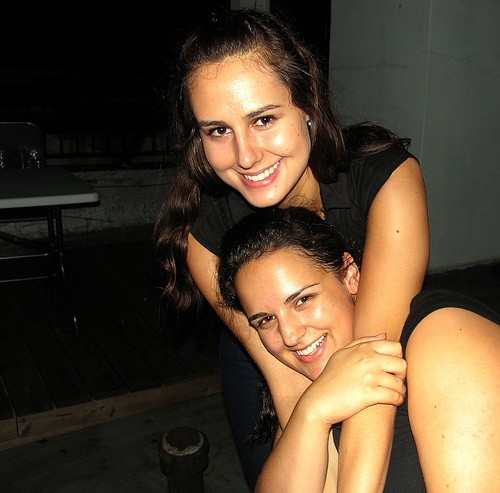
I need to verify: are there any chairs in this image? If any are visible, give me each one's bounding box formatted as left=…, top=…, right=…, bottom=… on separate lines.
left=0, top=122, right=80, bottom=308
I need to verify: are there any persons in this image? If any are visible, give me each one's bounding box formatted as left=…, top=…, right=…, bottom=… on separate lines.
left=214, top=206, right=500, bottom=492
left=152, top=7, right=429, bottom=493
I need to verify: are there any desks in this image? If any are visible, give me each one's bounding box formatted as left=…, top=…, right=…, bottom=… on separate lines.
left=0, top=166, right=99, bottom=342
